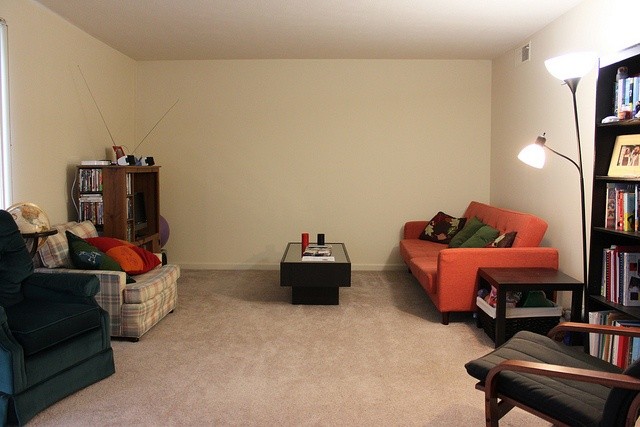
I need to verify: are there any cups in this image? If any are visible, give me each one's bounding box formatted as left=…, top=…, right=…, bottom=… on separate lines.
left=317, top=233, right=325, bottom=244
left=301, top=233, right=310, bottom=253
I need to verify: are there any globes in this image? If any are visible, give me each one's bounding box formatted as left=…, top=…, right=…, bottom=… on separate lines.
left=6, top=202, right=58, bottom=260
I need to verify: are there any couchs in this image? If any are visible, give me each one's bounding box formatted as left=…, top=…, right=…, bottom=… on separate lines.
left=399, top=199, right=560, bottom=324
left=26, top=220, right=181, bottom=341
left=0, top=210, right=116, bottom=427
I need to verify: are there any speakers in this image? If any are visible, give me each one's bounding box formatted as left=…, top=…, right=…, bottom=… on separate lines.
left=117, top=155, right=136, bottom=166
left=141, top=156, right=155, bottom=166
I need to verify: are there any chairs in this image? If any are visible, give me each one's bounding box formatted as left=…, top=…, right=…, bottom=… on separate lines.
left=465, top=321, right=640, bottom=427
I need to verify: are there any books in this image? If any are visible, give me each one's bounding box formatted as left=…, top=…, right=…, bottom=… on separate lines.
left=125, top=173, right=132, bottom=195
left=605, top=181, right=639, bottom=234
left=79, top=169, right=102, bottom=192
left=587, top=311, right=640, bottom=369
left=126, top=197, right=132, bottom=221
left=613, top=76, right=640, bottom=118
left=601, top=245, right=640, bottom=306
left=78, top=194, right=104, bottom=226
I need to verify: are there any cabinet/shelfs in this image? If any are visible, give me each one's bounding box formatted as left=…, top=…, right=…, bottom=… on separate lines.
left=75, top=163, right=161, bottom=256
left=583, top=41, right=638, bottom=362
left=476, top=267, right=584, bottom=349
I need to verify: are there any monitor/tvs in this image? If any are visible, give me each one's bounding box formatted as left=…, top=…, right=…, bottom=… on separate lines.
left=133, top=191, right=148, bottom=240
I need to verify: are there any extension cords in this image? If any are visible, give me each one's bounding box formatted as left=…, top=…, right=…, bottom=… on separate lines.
left=80, top=160, right=112, bottom=166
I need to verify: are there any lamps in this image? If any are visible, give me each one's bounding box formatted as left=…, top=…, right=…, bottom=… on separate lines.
left=514, top=49, right=599, bottom=318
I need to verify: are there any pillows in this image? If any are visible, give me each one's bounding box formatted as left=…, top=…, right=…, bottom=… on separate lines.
left=460, top=226, right=500, bottom=248
left=35, top=219, right=99, bottom=268
left=418, top=210, right=467, bottom=244
left=447, top=216, right=485, bottom=247
left=485, top=231, right=517, bottom=247
left=66, top=229, right=135, bottom=283
left=82, top=234, right=163, bottom=272
